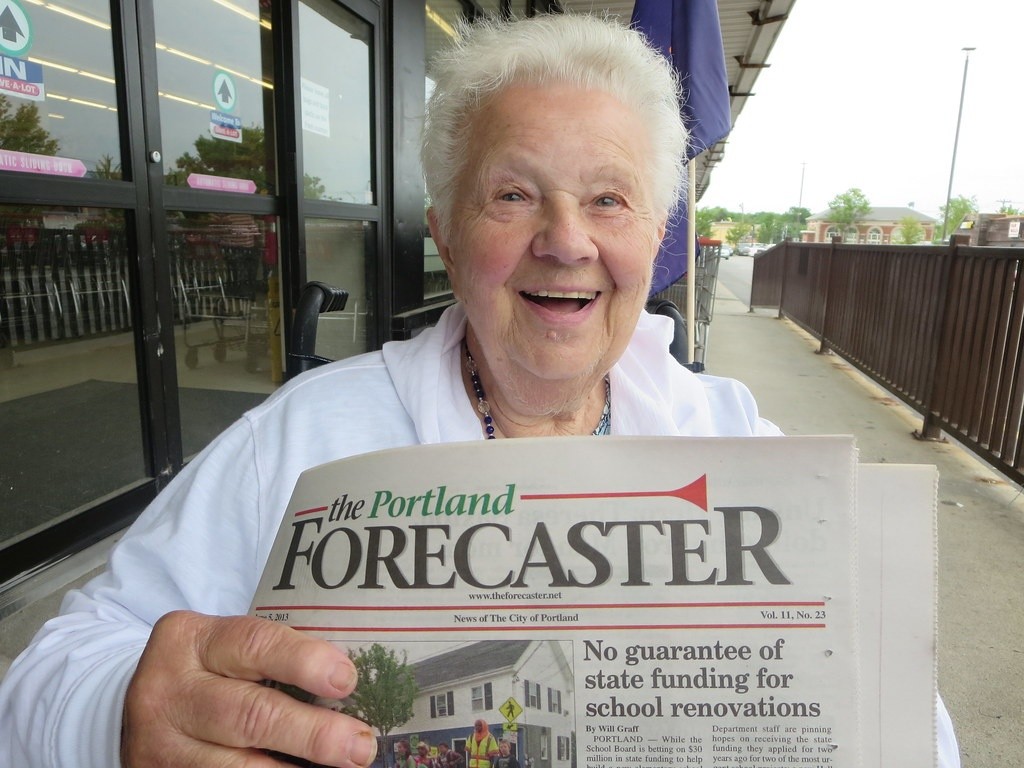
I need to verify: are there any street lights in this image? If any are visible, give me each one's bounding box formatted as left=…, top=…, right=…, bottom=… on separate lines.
left=795, top=162, right=811, bottom=236
left=940, top=46, right=977, bottom=241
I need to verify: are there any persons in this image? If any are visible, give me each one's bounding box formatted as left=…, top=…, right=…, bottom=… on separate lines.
left=464, top=720, right=500, bottom=768
left=395, top=740, right=428, bottom=768
left=434, top=743, right=464, bottom=768
left=493, top=739, right=521, bottom=768
left=208, top=213, right=259, bottom=302
left=0, top=13, right=958, bottom=768
left=416, top=742, right=436, bottom=768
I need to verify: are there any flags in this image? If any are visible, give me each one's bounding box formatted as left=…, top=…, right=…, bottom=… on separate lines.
left=628, top=0, right=731, bottom=301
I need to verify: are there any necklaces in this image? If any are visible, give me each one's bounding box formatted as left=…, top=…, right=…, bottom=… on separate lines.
left=466, top=344, right=495, bottom=440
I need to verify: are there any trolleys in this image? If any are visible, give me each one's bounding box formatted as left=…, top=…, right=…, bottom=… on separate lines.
left=648, top=237, right=722, bottom=373
left=289, top=278, right=697, bottom=377
left=169, top=255, right=271, bottom=374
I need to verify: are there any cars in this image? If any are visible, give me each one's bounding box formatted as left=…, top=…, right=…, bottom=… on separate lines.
left=714, top=241, right=777, bottom=260
